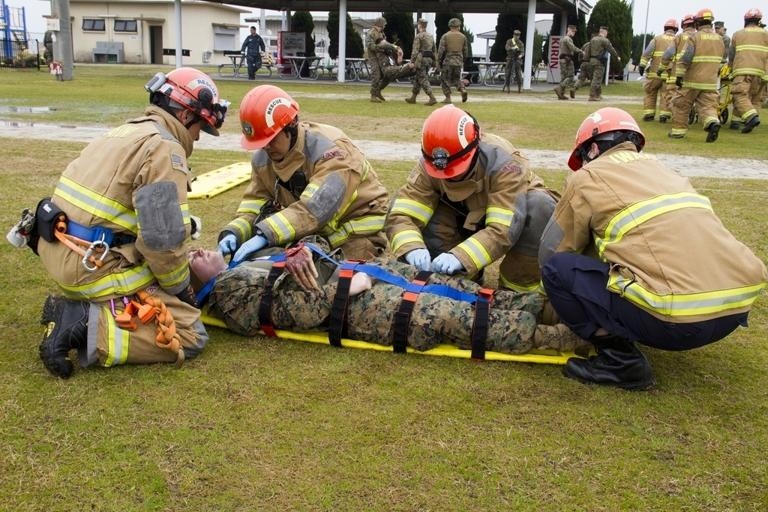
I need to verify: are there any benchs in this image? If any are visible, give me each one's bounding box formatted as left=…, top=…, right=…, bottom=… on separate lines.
left=216, top=52, right=508, bottom=88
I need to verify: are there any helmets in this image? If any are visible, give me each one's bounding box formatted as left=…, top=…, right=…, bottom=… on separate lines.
left=448, top=18, right=461, bottom=27
left=375, top=17, right=386, bottom=27
left=421, top=104, right=481, bottom=180
left=238, top=85, right=299, bottom=150
left=664, top=8, right=714, bottom=32
left=744, top=8, right=762, bottom=19
left=567, top=107, right=645, bottom=171
left=150, top=67, right=221, bottom=137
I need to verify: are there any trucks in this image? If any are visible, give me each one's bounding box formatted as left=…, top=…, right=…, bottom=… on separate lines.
left=259, top=37, right=279, bottom=64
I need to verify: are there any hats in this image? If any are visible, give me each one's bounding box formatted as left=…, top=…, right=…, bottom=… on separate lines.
left=715, top=22, right=724, bottom=27
left=417, top=18, right=428, bottom=27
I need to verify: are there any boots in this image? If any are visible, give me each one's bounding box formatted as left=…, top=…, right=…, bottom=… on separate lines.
left=38, top=294, right=90, bottom=380
left=405, top=93, right=417, bottom=104
left=570, top=87, right=577, bottom=98
left=706, top=123, right=720, bottom=142
left=460, top=88, right=467, bottom=102
left=563, top=332, right=657, bottom=392
left=425, top=95, right=437, bottom=106
left=441, top=94, right=451, bottom=103
left=554, top=86, right=567, bottom=99
left=741, top=116, right=760, bottom=134
left=371, top=94, right=381, bottom=102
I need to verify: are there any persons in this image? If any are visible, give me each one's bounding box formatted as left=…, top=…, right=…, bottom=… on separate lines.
left=241, top=26, right=265, bottom=80
left=554, top=25, right=584, bottom=100
left=187, top=239, right=594, bottom=354
left=365, top=18, right=391, bottom=103
left=585, top=26, right=621, bottom=101
left=375, top=38, right=417, bottom=81
left=639, top=8, right=768, bottom=143
left=438, top=18, right=468, bottom=104
left=405, top=19, right=437, bottom=106
left=217, top=86, right=390, bottom=267
left=570, top=34, right=597, bottom=98
left=15, top=66, right=230, bottom=380
left=505, top=30, right=525, bottom=93
left=539, top=108, right=766, bottom=392
left=385, top=103, right=558, bottom=293
left=428, top=76, right=470, bottom=87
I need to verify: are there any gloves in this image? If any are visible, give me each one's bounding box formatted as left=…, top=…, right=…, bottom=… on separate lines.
left=639, top=66, right=644, bottom=77
left=233, top=234, right=268, bottom=263
left=431, top=252, right=463, bottom=275
left=218, top=234, right=237, bottom=255
left=405, top=248, right=431, bottom=272
left=657, top=70, right=663, bottom=77
left=676, top=76, right=684, bottom=87
